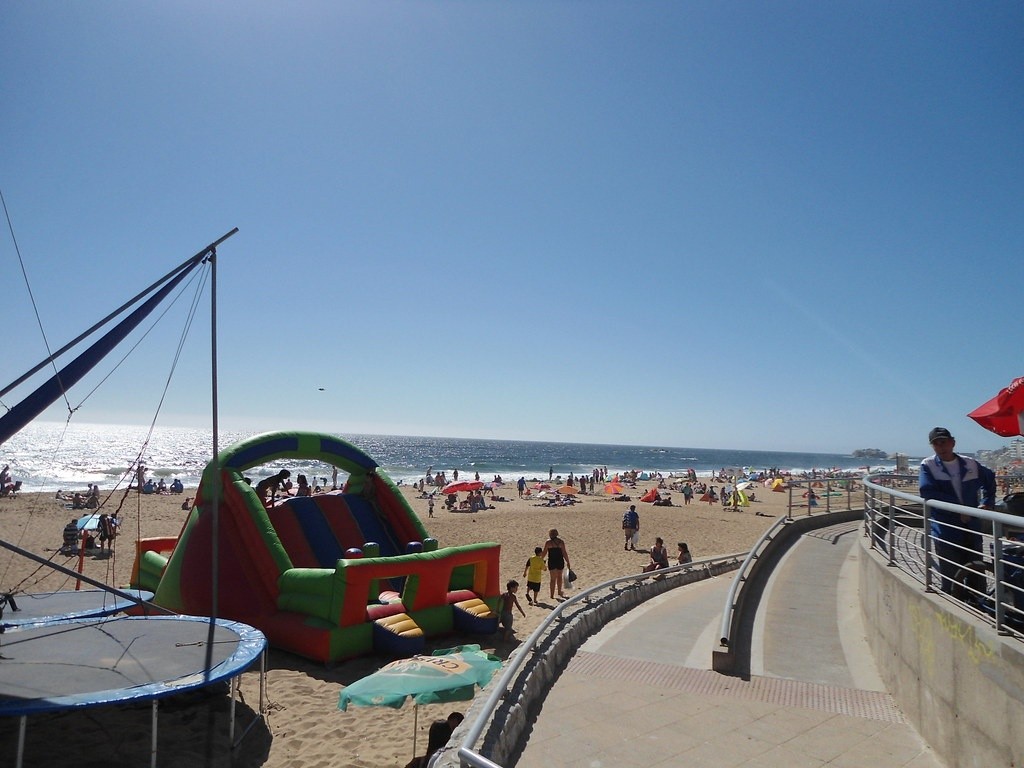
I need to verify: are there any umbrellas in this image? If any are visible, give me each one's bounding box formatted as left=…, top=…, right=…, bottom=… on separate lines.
left=611, top=476, right=619, bottom=483
left=76, top=514, right=118, bottom=532
left=559, top=486, right=578, bottom=497
left=966, top=376, right=1024, bottom=437
left=765, top=478, right=773, bottom=487
left=535, top=484, right=551, bottom=489
left=442, top=480, right=469, bottom=502
left=641, top=487, right=658, bottom=502
left=604, top=483, right=624, bottom=493
left=459, top=481, right=484, bottom=492
left=858, top=466, right=866, bottom=469
left=732, top=482, right=751, bottom=491
left=338, top=644, right=503, bottom=760
left=771, top=479, right=782, bottom=488
left=674, top=478, right=689, bottom=483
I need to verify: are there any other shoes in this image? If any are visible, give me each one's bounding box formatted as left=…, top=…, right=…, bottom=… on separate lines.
left=625, top=543, right=634, bottom=551
left=526, top=594, right=532, bottom=602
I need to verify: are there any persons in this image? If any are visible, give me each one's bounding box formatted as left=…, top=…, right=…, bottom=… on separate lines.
left=63, top=513, right=120, bottom=555
left=497, top=580, right=527, bottom=642
left=427, top=712, right=465, bottom=761
left=650, top=537, right=670, bottom=575
left=428, top=494, right=435, bottom=518
left=676, top=542, right=693, bottom=572
left=540, top=528, right=570, bottom=599
left=395, top=463, right=1024, bottom=512
left=136, top=466, right=183, bottom=494
left=182, top=498, right=191, bottom=510
left=622, top=505, right=639, bottom=550
left=55, top=483, right=100, bottom=509
left=245, top=465, right=345, bottom=510
left=919, top=427, right=997, bottom=612
left=0, top=466, right=10, bottom=492
left=523, top=547, right=547, bottom=602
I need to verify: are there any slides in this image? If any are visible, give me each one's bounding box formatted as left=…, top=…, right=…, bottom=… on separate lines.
left=264, top=491, right=408, bottom=593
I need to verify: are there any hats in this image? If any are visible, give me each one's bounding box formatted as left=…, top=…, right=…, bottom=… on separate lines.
left=928, top=426, right=953, bottom=443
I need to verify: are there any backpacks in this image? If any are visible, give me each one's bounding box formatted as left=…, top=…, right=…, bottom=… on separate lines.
left=622, top=511, right=637, bottom=528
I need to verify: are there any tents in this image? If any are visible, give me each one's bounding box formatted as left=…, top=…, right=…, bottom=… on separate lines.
left=637, top=472, right=648, bottom=480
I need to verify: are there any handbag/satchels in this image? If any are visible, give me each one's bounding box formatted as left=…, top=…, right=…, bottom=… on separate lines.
left=563, top=566, right=577, bottom=589
left=632, top=530, right=639, bottom=544
